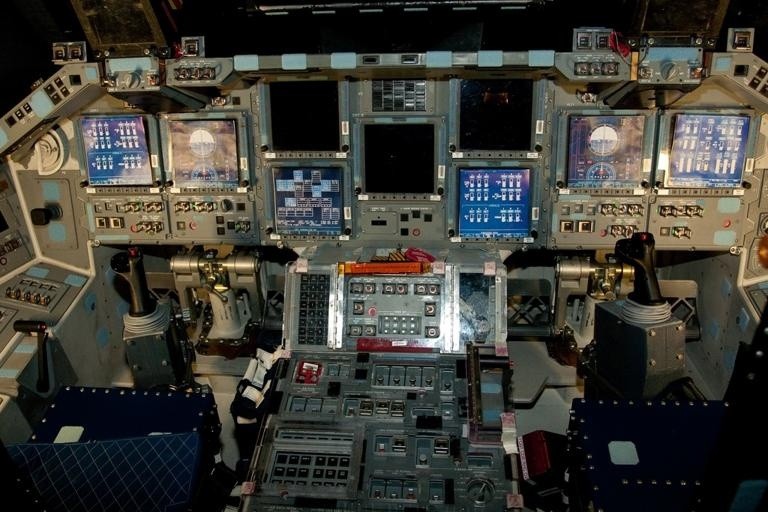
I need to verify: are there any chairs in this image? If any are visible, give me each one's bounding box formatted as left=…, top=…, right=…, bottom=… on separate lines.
left=562, top=302, right=768, bottom=511
left=0, top=382, right=235, bottom=511
